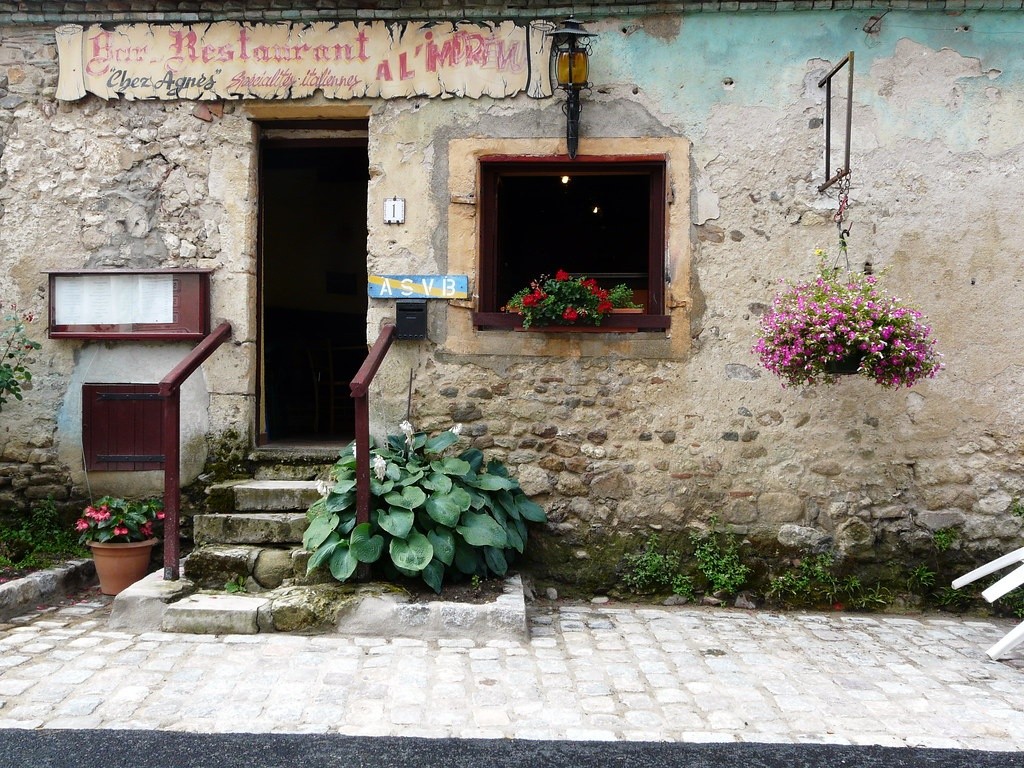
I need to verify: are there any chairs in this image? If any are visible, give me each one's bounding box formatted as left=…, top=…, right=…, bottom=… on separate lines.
left=304, top=336, right=352, bottom=433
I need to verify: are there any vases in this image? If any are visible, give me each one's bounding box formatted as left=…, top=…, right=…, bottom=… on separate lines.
left=82, top=538, right=159, bottom=596
left=821, top=337, right=865, bottom=376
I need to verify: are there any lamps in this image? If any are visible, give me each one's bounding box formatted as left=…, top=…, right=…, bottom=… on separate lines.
left=544, top=14, right=598, bottom=158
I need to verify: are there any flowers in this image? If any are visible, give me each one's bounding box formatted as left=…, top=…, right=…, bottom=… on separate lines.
left=499, top=268, right=636, bottom=329
left=75, top=495, right=168, bottom=544
left=749, top=250, right=945, bottom=393
